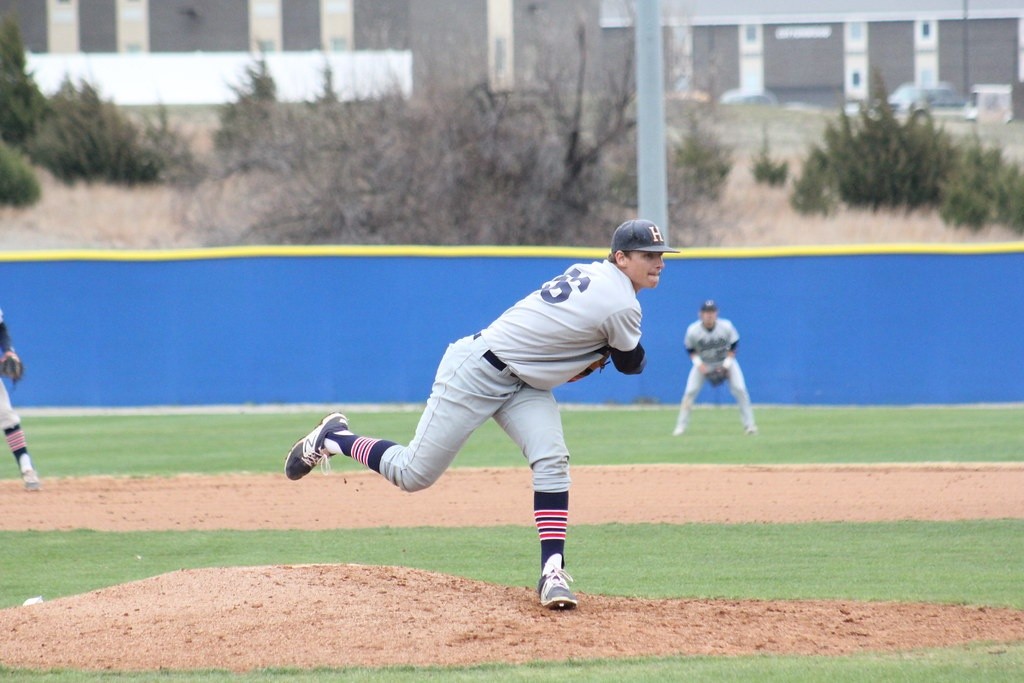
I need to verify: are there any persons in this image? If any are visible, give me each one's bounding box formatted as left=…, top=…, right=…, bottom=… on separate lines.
left=0, top=309, right=40, bottom=491
left=284, top=220, right=680, bottom=609
left=672, top=300, right=759, bottom=436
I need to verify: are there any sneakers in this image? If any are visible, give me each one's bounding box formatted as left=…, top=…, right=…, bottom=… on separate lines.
left=284, top=412, right=348, bottom=481
left=538, top=560, right=577, bottom=609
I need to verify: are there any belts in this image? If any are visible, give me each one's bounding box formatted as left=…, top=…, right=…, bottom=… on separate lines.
left=473, top=332, right=518, bottom=378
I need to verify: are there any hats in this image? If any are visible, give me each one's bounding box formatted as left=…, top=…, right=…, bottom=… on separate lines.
left=610, top=219, right=681, bottom=253
left=702, top=301, right=716, bottom=312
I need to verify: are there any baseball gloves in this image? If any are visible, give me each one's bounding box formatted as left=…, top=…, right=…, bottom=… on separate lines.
left=704, top=368, right=730, bottom=386
left=567, top=349, right=610, bottom=382
left=0, top=351, right=24, bottom=381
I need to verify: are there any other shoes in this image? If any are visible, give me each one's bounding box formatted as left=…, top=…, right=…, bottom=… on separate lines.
left=21, top=469, right=41, bottom=491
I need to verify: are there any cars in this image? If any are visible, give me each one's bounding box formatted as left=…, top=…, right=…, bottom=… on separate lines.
left=887, top=82, right=966, bottom=115
left=720, top=89, right=778, bottom=108
